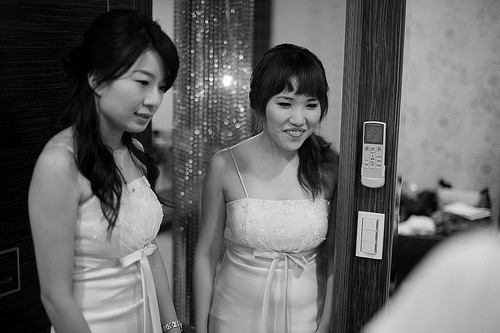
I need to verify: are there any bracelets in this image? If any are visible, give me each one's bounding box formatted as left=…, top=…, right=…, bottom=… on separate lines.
left=163, top=321, right=183, bottom=332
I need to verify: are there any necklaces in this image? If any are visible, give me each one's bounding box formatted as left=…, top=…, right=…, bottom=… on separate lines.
left=110, top=143, right=135, bottom=192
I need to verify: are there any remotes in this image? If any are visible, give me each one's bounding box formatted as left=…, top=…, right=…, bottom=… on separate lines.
left=361, top=121, right=387, bottom=188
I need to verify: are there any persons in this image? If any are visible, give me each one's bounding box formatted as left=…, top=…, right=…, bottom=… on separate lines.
left=28, top=7, right=182, bottom=333
left=192, top=43, right=339, bottom=333
